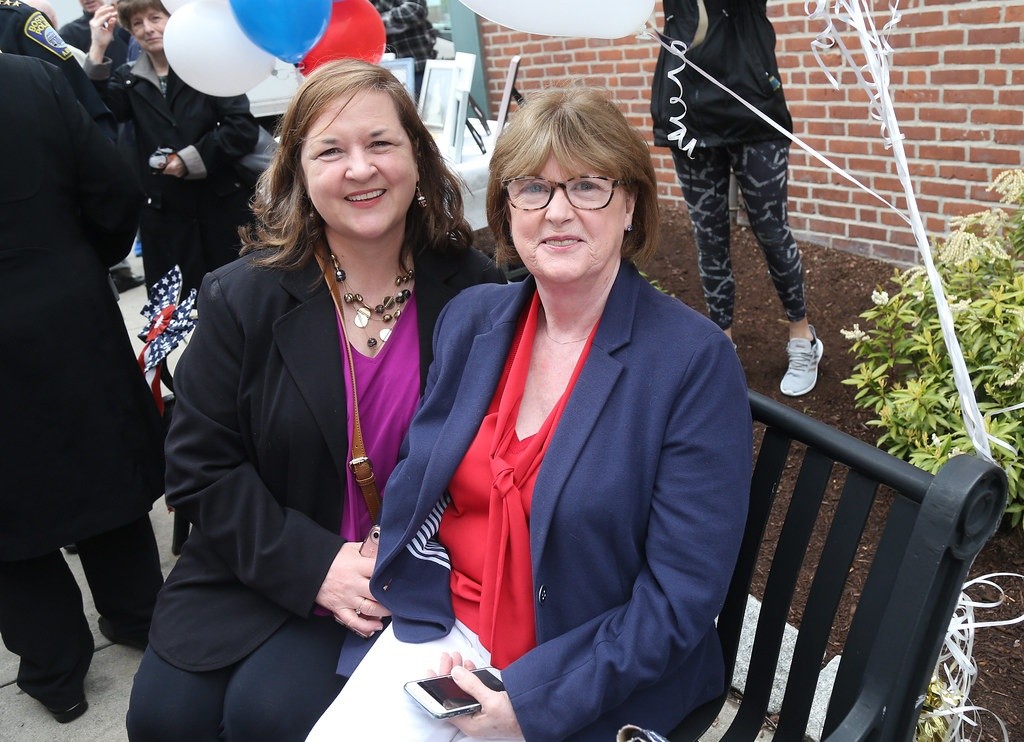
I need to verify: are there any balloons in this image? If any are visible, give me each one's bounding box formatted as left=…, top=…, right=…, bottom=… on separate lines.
left=459, top=1, right=658, bottom=39
left=163, top=0, right=386, bottom=98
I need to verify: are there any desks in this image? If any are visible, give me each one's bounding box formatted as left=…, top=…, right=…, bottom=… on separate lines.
left=439, top=119, right=511, bottom=228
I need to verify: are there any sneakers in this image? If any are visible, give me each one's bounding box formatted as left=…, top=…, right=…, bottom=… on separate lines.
left=780, top=325, right=823, bottom=396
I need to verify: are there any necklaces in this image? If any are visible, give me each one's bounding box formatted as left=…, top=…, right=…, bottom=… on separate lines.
left=331, top=252, right=414, bottom=350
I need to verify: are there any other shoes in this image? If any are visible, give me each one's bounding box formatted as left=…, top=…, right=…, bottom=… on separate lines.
left=48, top=692, right=88, bottom=724
left=98, top=614, right=148, bottom=651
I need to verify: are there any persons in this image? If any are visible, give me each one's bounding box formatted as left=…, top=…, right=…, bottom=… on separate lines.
left=0, top=0, right=119, bottom=147
left=84, top=0, right=260, bottom=308
left=58, top=0, right=131, bottom=71
left=0, top=53, right=167, bottom=723
left=305, top=90, right=751, bottom=742
left=127, top=61, right=506, bottom=742
left=649, top=0, right=823, bottom=397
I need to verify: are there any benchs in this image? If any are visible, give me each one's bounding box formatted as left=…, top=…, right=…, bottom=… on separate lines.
left=160, top=359, right=1009, bottom=742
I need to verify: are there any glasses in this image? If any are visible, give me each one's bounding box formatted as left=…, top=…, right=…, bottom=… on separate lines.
left=501, top=173, right=634, bottom=211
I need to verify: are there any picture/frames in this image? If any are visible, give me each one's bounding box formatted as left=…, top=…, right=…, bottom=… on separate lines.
left=381, top=50, right=476, bottom=163
left=496, top=55, right=523, bottom=137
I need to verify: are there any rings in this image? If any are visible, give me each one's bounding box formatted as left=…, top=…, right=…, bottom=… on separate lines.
left=356, top=599, right=365, bottom=614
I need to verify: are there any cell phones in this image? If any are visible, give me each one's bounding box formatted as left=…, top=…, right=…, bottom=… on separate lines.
left=405, top=667, right=507, bottom=718
left=335, top=526, right=383, bottom=638
